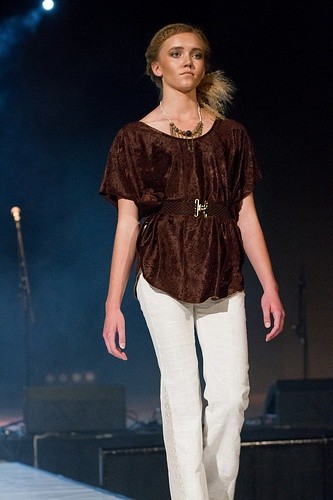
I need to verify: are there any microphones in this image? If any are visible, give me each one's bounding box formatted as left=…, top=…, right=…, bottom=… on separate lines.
left=11, top=206, right=20, bottom=222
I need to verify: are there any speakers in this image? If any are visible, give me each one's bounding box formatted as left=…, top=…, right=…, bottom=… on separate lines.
left=23, top=383, right=126, bottom=435
left=262, top=378, right=333, bottom=428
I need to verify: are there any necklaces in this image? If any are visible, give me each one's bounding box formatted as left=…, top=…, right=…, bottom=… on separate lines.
left=159, top=98, right=206, bottom=138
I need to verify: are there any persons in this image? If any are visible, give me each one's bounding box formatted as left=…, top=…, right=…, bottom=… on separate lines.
left=99, top=21, right=287, bottom=499
